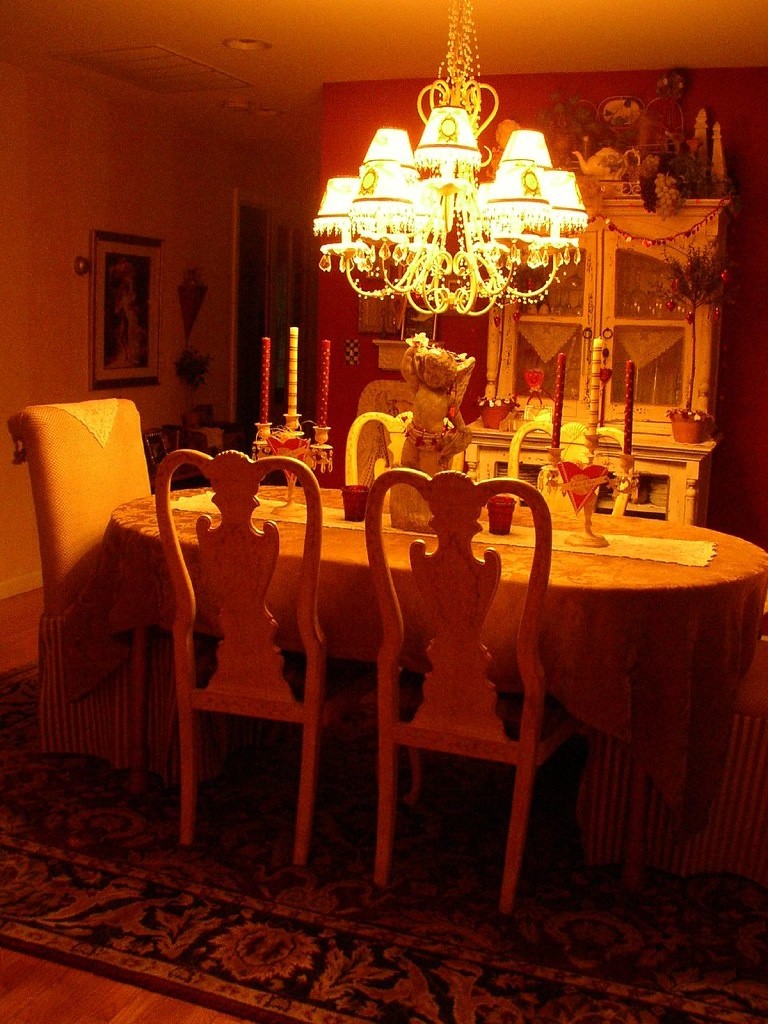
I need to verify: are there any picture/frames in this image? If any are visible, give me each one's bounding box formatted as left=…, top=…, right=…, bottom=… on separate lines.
left=146, top=427, right=166, bottom=468
left=89, top=229, right=163, bottom=389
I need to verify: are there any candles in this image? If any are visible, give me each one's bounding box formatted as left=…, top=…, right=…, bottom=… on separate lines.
left=252, top=321, right=333, bottom=428
left=591, top=332, right=603, bottom=435
left=623, top=360, right=637, bottom=455
left=553, top=350, right=567, bottom=448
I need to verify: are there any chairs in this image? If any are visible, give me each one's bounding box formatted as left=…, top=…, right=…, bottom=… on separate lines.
left=507, top=419, right=636, bottom=515
left=6, top=399, right=152, bottom=793
left=345, top=404, right=428, bottom=515
left=580, top=609, right=766, bottom=888
left=366, top=472, right=563, bottom=912
left=132, top=449, right=318, bottom=865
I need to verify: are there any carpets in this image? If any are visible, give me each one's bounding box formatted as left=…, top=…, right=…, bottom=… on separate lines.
left=0, top=627, right=767, bottom=1023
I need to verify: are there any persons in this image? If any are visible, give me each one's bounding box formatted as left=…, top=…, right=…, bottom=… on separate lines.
left=400, top=345, right=472, bottom=477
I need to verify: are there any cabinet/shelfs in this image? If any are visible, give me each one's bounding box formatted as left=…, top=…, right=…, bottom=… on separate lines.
left=474, top=435, right=720, bottom=519
left=487, top=197, right=730, bottom=436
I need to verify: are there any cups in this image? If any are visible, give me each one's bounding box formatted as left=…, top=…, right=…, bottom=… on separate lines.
left=340, top=485, right=369, bottom=522
left=487, top=495, right=516, bottom=535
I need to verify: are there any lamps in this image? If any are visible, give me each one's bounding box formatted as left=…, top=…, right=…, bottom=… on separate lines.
left=311, top=0, right=589, bottom=317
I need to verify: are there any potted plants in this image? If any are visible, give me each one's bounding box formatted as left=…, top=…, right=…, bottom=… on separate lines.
left=176, top=348, right=214, bottom=427
left=667, top=226, right=728, bottom=442
left=483, top=229, right=520, bottom=431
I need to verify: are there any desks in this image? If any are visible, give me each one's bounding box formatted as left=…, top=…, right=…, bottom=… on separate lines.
left=101, top=483, right=766, bottom=882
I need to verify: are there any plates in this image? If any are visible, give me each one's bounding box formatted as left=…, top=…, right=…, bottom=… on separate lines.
left=496, top=463, right=540, bottom=489
left=599, top=474, right=667, bottom=509
